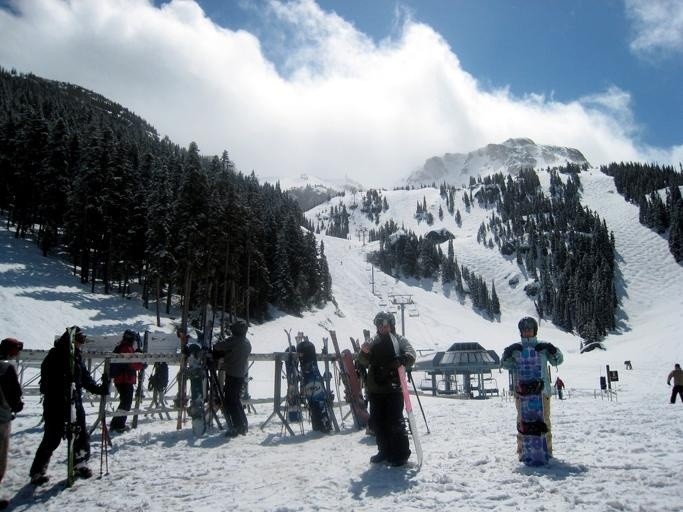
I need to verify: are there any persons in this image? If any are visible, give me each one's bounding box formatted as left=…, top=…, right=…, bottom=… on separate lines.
left=357, top=312, right=416, bottom=468
left=0, top=338, right=25, bottom=510
left=667, top=363, right=683, bottom=404
left=29, top=325, right=109, bottom=485
left=500, top=317, right=564, bottom=462
left=102, top=328, right=148, bottom=433
left=553, top=376, right=566, bottom=400
left=213, top=321, right=251, bottom=437
left=152, top=362, right=169, bottom=409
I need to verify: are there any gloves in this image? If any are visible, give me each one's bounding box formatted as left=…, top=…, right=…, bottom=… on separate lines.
left=393, top=354, right=410, bottom=367
left=98, top=383, right=108, bottom=394
left=64, top=390, right=77, bottom=403
left=535, top=342, right=555, bottom=354
left=504, top=343, right=521, bottom=357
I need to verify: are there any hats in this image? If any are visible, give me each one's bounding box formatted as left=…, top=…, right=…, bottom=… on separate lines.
left=123, top=329, right=138, bottom=345
left=230, top=320, right=247, bottom=335
left=63, top=326, right=83, bottom=346
left=0, top=339, right=18, bottom=357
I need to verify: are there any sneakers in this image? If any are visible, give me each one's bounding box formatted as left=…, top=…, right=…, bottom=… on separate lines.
left=226, top=424, right=248, bottom=436
left=110, top=425, right=129, bottom=433
left=32, top=473, right=48, bottom=485
left=370, top=449, right=407, bottom=465
left=73, top=466, right=91, bottom=478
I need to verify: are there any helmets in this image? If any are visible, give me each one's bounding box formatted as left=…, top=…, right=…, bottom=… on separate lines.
left=518, top=317, right=537, bottom=335
left=375, top=311, right=394, bottom=327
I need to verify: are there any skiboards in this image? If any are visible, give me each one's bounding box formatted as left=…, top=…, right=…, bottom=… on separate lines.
left=131, top=331, right=148, bottom=428
left=280, top=329, right=370, bottom=436
left=67, top=326, right=78, bottom=488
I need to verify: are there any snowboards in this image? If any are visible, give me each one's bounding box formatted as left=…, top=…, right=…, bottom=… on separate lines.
left=515, top=343, right=549, bottom=467
left=389, top=332, right=423, bottom=470
left=187, top=303, right=214, bottom=436
